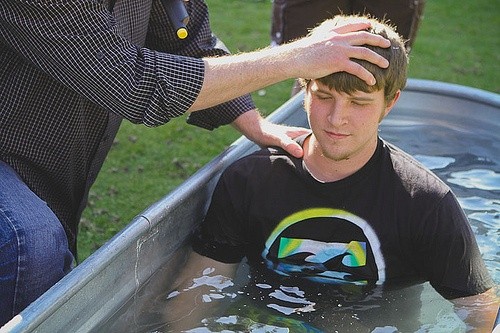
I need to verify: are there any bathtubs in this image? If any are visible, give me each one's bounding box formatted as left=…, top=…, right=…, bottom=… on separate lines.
left=0, top=75, right=499, bottom=333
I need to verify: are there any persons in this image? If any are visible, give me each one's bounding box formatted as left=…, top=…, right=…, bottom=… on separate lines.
left=0, top=0, right=393, bottom=328
left=164, top=13, right=500, bottom=333
